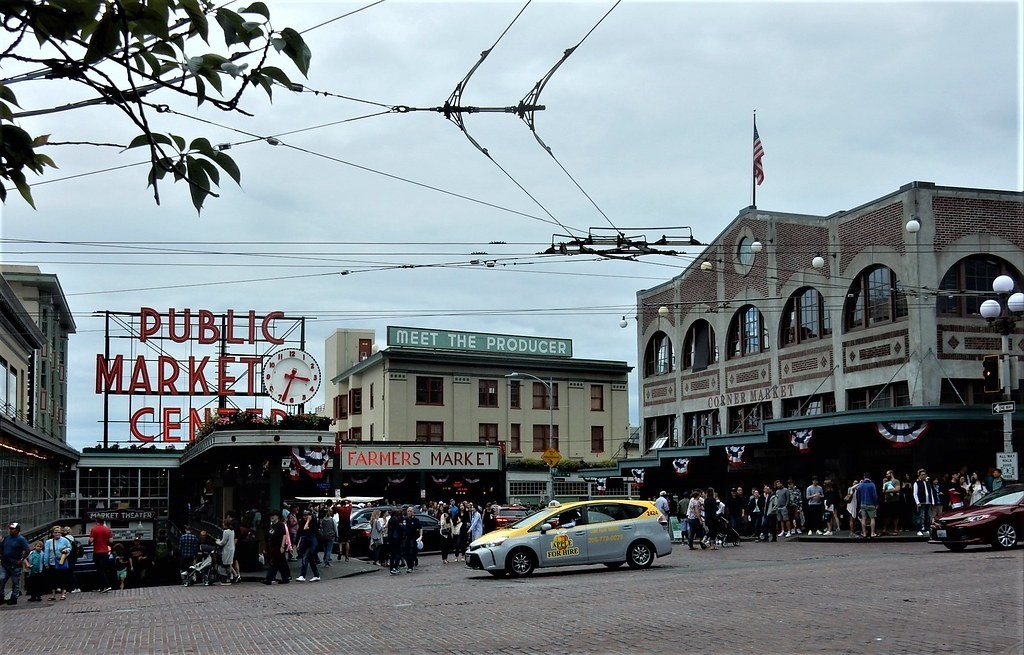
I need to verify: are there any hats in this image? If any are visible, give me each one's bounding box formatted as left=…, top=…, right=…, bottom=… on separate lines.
left=8, top=523, right=20, bottom=532
left=302, top=509, right=313, bottom=515
left=660, top=491, right=668, bottom=495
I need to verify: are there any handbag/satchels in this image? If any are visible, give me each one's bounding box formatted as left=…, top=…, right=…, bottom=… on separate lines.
left=452, top=522, right=463, bottom=535
left=844, top=490, right=856, bottom=503
left=55, top=557, right=68, bottom=569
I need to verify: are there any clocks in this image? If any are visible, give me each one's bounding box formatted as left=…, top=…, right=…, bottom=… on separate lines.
left=262, top=348, right=321, bottom=405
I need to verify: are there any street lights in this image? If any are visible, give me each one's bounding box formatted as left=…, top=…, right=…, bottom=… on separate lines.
left=504, top=371, right=553, bottom=502
left=980, top=275, right=1024, bottom=452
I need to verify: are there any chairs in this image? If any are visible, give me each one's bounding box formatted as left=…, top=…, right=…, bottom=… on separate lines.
left=569, top=509, right=579, bottom=520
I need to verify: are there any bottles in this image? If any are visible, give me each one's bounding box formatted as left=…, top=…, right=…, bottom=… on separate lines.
left=59, top=552, right=66, bottom=565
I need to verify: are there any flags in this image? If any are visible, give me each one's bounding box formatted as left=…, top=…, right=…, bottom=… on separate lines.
left=753, top=115, right=765, bottom=186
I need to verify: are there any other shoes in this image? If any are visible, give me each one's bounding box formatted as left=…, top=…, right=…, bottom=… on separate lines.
left=682, top=527, right=931, bottom=551
left=28, top=598, right=36, bottom=602
left=60, top=597, right=66, bottom=600
left=48, top=598, right=56, bottom=601
left=36, top=598, right=41, bottom=601
left=92, top=588, right=101, bottom=592
left=7, top=598, right=17, bottom=605
left=220, top=556, right=466, bottom=586
left=0, top=596, right=4, bottom=605
left=102, top=586, right=111, bottom=592
left=71, top=588, right=81, bottom=594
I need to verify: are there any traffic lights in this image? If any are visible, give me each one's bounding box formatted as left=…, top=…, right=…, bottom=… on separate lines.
left=982, top=355, right=1000, bottom=392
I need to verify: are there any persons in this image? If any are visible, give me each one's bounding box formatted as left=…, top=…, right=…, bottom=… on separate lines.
left=1, top=494, right=544, bottom=607
left=657, top=469, right=1004, bottom=551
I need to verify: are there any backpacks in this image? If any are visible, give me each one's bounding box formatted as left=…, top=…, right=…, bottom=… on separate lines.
left=65, top=536, right=84, bottom=558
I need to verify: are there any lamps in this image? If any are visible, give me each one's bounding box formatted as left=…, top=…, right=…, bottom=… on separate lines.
left=906, top=215, right=922, bottom=234
left=619, top=315, right=639, bottom=328
left=812, top=251, right=837, bottom=267
left=701, top=257, right=722, bottom=272
left=751, top=238, right=774, bottom=253
left=658, top=303, right=679, bottom=317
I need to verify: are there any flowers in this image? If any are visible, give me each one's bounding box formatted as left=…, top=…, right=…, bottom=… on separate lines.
left=215, top=411, right=330, bottom=425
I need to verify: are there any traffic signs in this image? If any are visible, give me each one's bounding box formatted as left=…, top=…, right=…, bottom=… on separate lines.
left=992, top=401, right=1016, bottom=415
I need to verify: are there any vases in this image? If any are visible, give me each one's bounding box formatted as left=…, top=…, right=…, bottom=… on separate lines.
left=216, top=424, right=329, bottom=431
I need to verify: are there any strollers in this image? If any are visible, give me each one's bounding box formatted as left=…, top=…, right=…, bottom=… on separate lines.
left=179, top=544, right=223, bottom=586
left=716, top=512, right=744, bottom=548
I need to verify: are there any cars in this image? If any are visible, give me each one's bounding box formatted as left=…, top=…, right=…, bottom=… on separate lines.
left=929, top=483, right=1024, bottom=551
left=491, top=504, right=530, bottom=529
left=72, top=545, right=115, bottom=589
left=342, top=506, right=441, bottom=552
left=465, top=500, right=673, bottom=579
